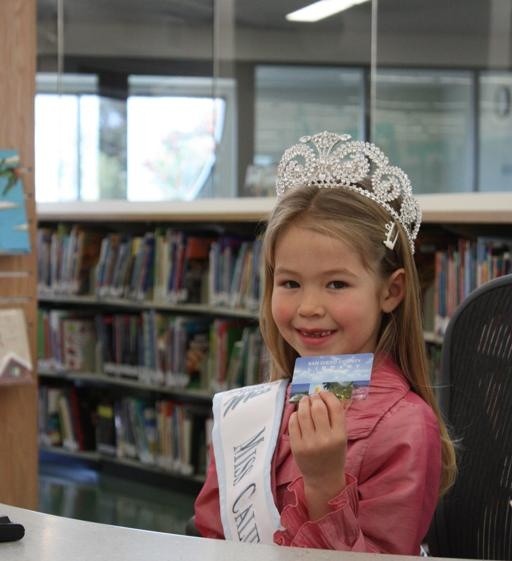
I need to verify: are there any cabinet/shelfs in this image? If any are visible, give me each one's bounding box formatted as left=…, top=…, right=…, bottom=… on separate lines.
left=38, top=193, right=512, bottom=498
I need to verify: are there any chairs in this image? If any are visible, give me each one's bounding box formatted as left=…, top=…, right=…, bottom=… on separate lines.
left=430, top=272, right=510, bottom=558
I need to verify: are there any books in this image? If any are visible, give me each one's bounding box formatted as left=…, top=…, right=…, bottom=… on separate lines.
left=424, top=235, right=512, bottom=395
left=36, top=225, right=281, bottom=484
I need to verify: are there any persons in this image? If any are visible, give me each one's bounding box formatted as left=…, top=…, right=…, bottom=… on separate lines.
left=193, top=179, right=454, bottom=558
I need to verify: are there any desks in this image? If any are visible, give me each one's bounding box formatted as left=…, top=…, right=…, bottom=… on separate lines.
left=0, top=497, right=418, bottom=559
